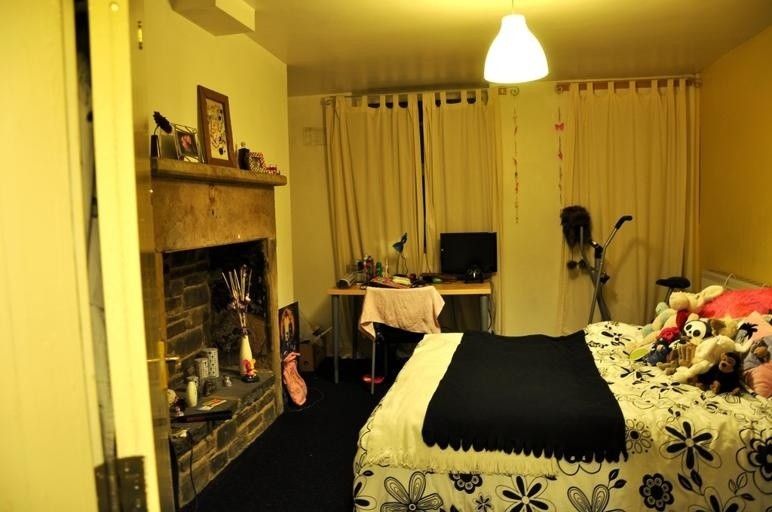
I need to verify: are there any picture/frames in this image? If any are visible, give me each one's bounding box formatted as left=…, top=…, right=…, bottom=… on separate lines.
left=197, top=85, right=237, bottom=167
left=176, top=131, right=200, bottom=161
left=249, top=152, right=265, bottom=172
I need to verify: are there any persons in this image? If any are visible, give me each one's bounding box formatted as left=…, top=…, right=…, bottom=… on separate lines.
left=181, top=132, right=196, bottom=153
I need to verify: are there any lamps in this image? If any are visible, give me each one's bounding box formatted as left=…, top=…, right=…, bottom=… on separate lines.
left=482, top=1, right=549, bottom=84
left=394, top=234, right=409, bottom=279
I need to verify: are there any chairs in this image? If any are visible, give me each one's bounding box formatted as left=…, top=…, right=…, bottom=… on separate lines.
left=365, top=285, right=436, bottom=395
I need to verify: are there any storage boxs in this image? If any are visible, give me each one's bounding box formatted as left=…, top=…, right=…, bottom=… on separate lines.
left=299, top=340, right=324, bottom=372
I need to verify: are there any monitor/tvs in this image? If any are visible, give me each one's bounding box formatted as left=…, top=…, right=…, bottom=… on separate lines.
left=440, top=232, right=498, bottom=284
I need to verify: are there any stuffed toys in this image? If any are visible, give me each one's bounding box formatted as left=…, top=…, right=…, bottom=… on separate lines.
left=642, top=337, right=672, bottom=366
left=656, top=313, right=738, bottom=375
left=693, top=352, right=742, bottom=395
left=626, top=285, right=723, bottom=361
left=672, top=316, right=753, bottom=382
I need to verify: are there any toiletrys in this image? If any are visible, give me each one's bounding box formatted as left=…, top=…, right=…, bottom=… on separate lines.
left=376, top=262, right=381, bottom=275
left=385, top=258, right=389, bottom=277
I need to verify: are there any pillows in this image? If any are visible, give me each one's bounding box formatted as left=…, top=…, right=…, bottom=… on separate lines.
left=732, top=311, right=772, bottom=398
left=350, top=322, right=772, bottom=512
left=700, top=288, right=772, bottom=319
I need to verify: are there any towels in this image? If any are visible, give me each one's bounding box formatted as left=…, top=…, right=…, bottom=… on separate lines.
left=357, top=286, right=445, bottom=342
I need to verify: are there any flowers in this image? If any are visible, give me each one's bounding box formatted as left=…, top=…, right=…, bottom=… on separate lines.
left=151, top=111, right=172, bottom=135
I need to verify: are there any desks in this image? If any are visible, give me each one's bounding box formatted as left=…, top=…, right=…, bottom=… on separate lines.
left=328, top=277, right=490, bottom=385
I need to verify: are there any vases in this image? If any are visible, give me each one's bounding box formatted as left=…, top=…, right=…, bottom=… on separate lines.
left=239, top=143, right=249, bottom=169
left=151, top=135, right=160, bottom=158
left=240, top=326, right=256, bottom=381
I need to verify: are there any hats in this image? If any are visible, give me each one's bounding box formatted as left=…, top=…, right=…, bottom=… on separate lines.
left=560, top=206, right=591, bottom=270
